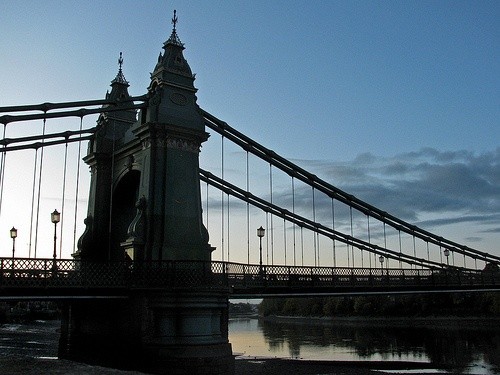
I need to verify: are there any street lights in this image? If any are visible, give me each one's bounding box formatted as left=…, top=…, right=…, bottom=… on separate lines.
left=10, top=226, right=18, bottom=277
left=52, top=208, right=60, bottom=271
left=256, top=226, right=265, bottom=278
left=380, top=255, right=384, bottom=279
left=444, top=249, right=450, bottom=268
